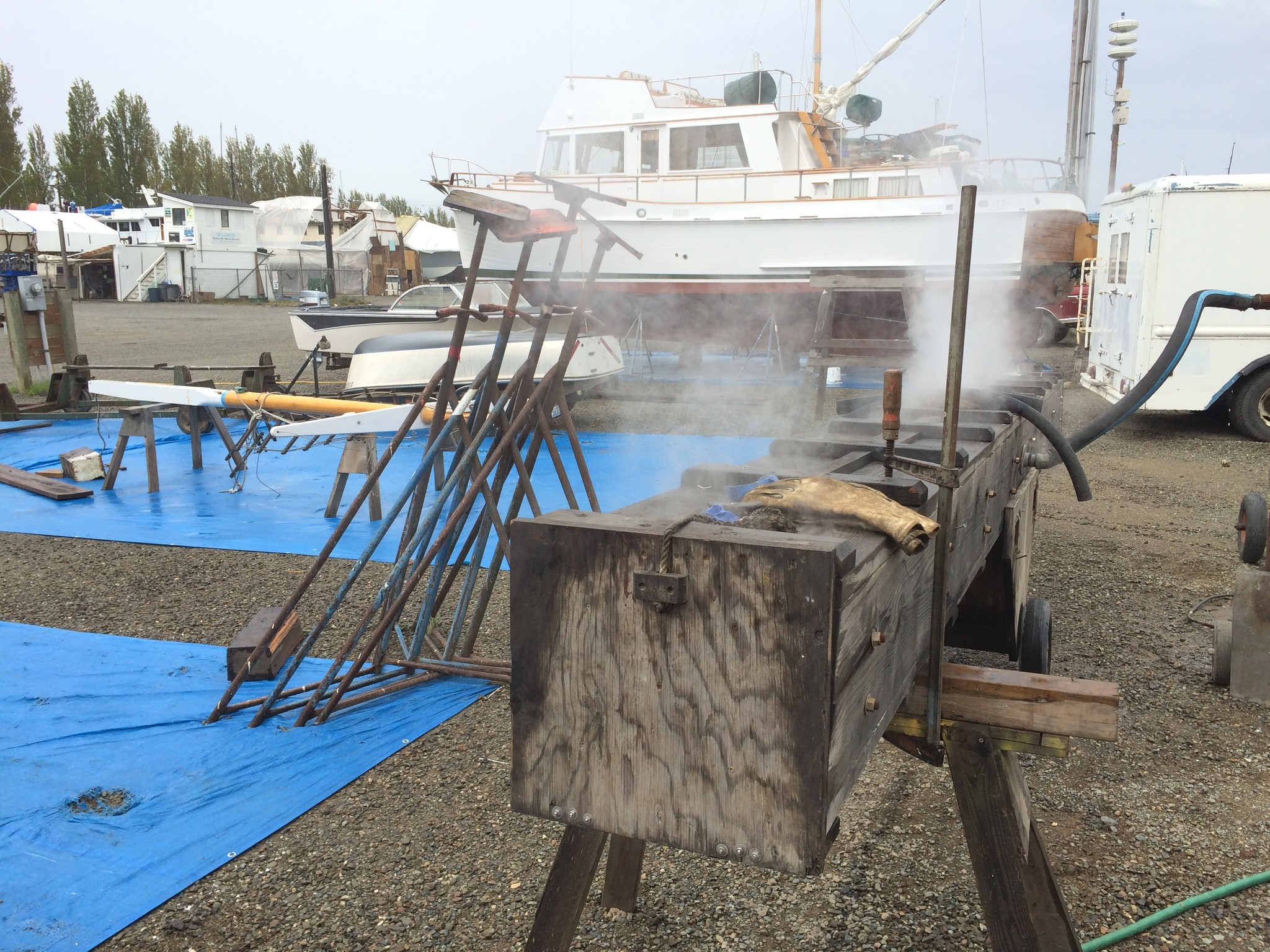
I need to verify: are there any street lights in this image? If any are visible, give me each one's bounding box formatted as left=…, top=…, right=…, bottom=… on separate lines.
left=1105, top=11, right=1140, bottom=197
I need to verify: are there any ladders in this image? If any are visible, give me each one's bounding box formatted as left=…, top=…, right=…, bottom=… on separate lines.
left=386, top=268, right=406, bottom=297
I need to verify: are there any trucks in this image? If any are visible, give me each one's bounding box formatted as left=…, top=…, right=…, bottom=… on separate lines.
left=1075, top=173, right=1270, bottom=443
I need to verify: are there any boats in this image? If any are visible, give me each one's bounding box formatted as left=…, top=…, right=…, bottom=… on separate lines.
left=287, top=279, right=595, bottom=371
left=341, top=331, right=626, bottom=416
left=416, top=0, right=1103, bottom=359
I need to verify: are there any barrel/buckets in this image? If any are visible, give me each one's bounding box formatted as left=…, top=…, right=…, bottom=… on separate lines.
left=825, top=367, right=843, bottom=384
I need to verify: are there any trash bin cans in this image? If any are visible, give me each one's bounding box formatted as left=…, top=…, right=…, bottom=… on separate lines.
left=148, top=288, right=161, bottom=303
left=157, top=284, right=179, bottom=302
